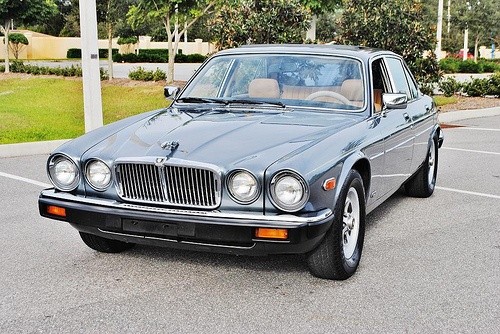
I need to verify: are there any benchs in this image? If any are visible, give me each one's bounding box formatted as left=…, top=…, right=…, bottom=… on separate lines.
left=280, top=84, right=383, bottom=112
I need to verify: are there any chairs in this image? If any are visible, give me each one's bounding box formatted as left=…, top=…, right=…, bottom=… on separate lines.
left=335, top=79, right=381, bottom=113
left=248, top=77, right=281, bottom=99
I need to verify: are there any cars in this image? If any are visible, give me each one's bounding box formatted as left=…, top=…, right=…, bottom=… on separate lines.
left=38, top=44, right=444, bottom=280
